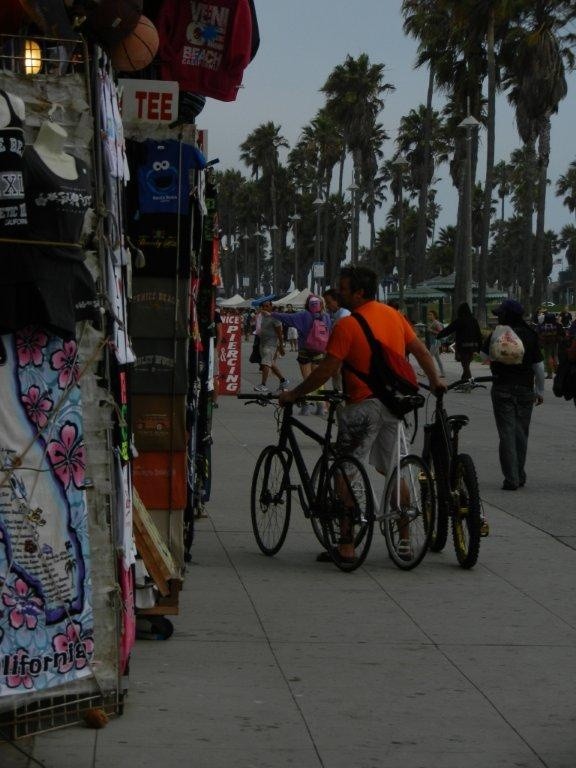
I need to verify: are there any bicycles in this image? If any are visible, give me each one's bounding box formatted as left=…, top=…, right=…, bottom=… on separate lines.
left=237, top=375, right=500, bottom=574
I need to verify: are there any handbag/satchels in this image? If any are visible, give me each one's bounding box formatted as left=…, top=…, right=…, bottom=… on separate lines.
left=371, top=338, right=420, bottom=396
left=489, top=325, right=525, bottom=366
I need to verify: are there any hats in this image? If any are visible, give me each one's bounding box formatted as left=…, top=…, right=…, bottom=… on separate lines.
left=491, top=299, right=525, bottom=322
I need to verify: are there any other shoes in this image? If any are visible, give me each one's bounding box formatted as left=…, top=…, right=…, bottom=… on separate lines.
left=315, top=405, right=325, bottom=415
left=298, top=404, right=311, bottom=416
left=254, top=384, right=268, bottom=391
left=316, top=547, right=359, bottom=564
left=278, top=378, right=290, bottom=393
left=395, top=536, right=414, bottom=562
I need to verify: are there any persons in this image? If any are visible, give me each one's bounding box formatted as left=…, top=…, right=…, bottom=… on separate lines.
left=436, top=302, right=483, bottom=381
left=216, top=286, right=419, bottom=419
left=415, top=309, right=445, bottom=379
left=480, top=298, right=546, bottom=489
left=278, top=264, right=449, bottom=564
left=526, top=303, right=576, bottom=408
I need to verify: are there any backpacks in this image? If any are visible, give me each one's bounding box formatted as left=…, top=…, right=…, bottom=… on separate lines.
left=304, top=311, right=330, bottom=354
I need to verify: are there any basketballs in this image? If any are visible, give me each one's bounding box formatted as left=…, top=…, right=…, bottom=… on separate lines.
left=110, top=15, right=158, bottom=70
